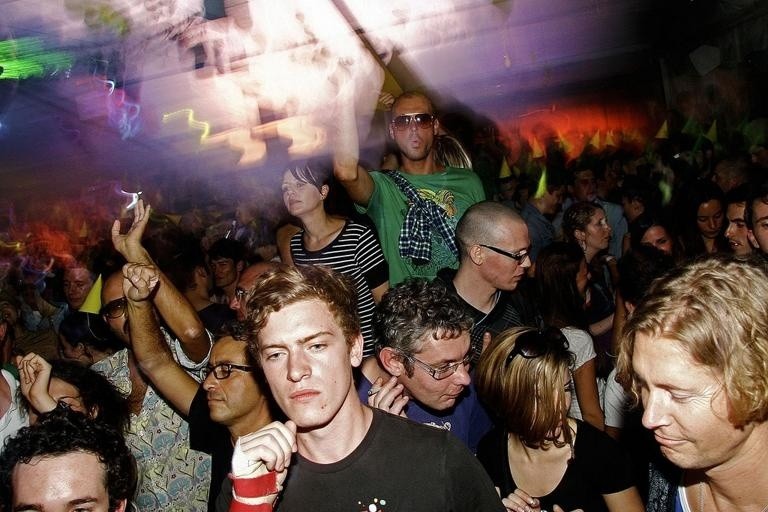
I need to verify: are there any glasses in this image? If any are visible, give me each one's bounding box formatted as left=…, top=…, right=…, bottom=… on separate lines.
left=100, top=296, right=128, bottom=318
left=479, top=244, right=532, bottom=264
left=398, top=346, right=476, bottom=379
left=235, top=289, right=250, bottom=298
left=199, top=363, right=254, bottom=379
left=504, top=327, right=569, bottom=369
left=390, top=113, right=435, bottom=129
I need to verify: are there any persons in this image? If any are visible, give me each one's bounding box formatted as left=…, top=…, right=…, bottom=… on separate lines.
left=355, top=280, right=494, bottom=457
left=0, top=178, right=299, bottom=510
left=223, top=267, right=505, bottom=511
left=480, top=241, right=768, bottom=510
left=471, top=71, right=768, bottom=300
left=326, top=56, right=484, bottom=286
left=435, top=199, right=531, bottom=381
left=283, top=161, right=387, bottom=355
